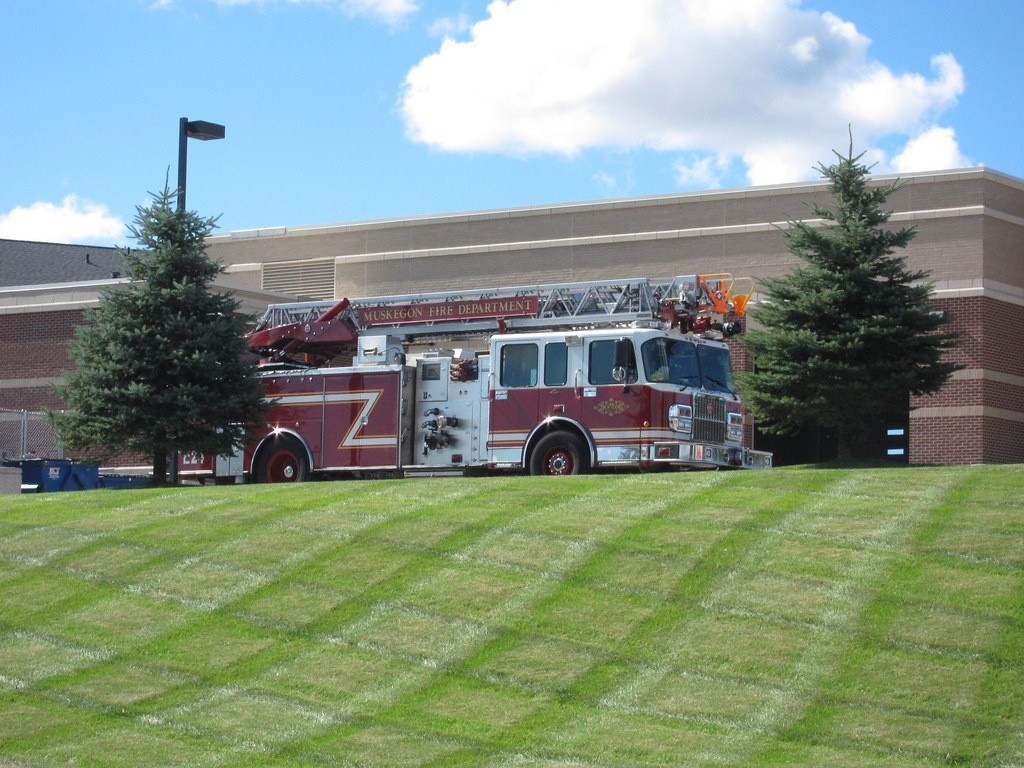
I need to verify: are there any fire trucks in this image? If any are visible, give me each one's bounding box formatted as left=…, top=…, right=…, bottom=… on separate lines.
left=167, top=273, right=773, bottom=485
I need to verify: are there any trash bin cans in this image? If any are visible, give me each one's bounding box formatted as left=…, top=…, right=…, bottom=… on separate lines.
left=21, top=459, right=99, bottom=493
left=95, top=474, right=152, bottom=488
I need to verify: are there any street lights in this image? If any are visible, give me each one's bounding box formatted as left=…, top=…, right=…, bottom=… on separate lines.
left=152, top=117, right=225, bottom=484
